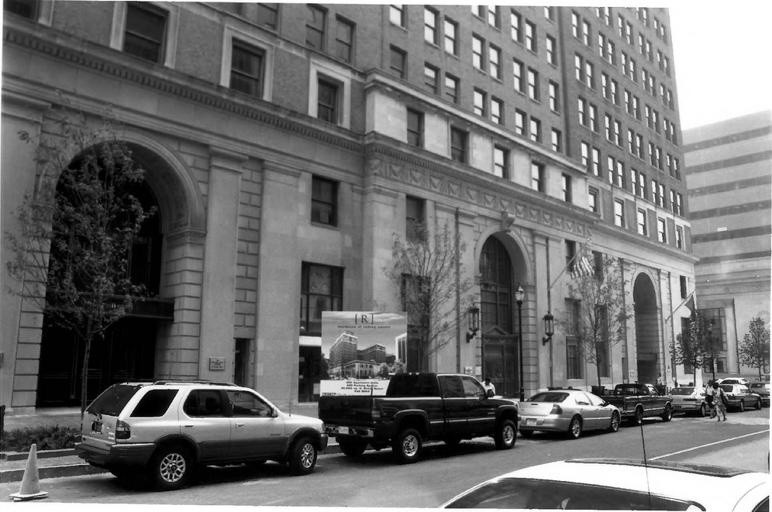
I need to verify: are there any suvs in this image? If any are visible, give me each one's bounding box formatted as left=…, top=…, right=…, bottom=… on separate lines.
left=75, top=380, right=329, bottom=486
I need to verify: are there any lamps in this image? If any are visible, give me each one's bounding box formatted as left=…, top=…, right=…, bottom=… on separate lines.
left=466, top=303, right=480, bottom=343
left=543, top=311, right=555, bottom=345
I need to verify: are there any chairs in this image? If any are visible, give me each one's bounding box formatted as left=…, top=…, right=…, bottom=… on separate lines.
left=206, top=398, right=219, bottom=412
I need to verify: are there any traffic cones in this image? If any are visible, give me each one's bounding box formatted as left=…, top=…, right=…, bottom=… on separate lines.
left=8, top=443, right=47, bottom=500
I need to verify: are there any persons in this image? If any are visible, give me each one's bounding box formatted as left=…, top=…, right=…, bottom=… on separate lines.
left=711, top=381, right=729, bottom=423
left=703, top=379, right=716, bottom=418
left=481, top=376, right=496, bottom=397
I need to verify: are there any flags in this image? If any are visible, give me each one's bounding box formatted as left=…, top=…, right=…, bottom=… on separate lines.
left=570, top=256, right=595, bottom=280
left=684, top=293, right=695, bottom=319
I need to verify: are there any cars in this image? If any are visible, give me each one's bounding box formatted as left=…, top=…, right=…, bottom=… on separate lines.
left=519, top=389, right=622, bottom=438
left=665, top=386, right=717, bottom=416
left=720, top=377, right=770, bottom=411
left=440, top=457, right=768, bottom=512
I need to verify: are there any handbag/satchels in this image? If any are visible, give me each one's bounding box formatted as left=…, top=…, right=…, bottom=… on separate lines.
left=720, top=388, right=729, bottom=407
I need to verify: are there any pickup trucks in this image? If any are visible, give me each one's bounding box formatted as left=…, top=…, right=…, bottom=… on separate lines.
left=602, top=385, right=673, bottom=424
left=319, top=370, right=521, bottom=460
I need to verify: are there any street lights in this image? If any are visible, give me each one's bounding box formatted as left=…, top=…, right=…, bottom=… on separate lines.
left=709, top=323, right=716, bottom=381
left=515, top=286, right=524, bottom=401
